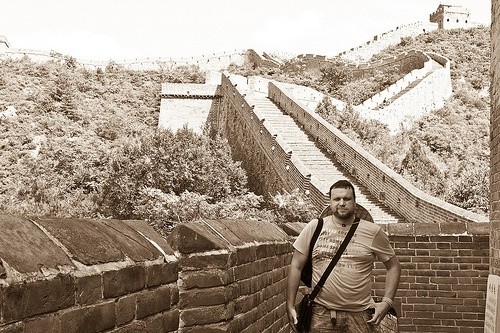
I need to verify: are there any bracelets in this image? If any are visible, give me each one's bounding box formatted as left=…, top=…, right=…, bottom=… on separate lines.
left=381, top=297, right=394, bottom=306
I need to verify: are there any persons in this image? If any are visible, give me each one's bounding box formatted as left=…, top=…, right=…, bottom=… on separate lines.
left=286, top=180, right=401, bottom=333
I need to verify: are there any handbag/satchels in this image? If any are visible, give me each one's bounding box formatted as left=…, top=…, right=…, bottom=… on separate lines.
left=296, top=294, right=313, bottom=332
left=300, top=217, right=323, bottom=288
